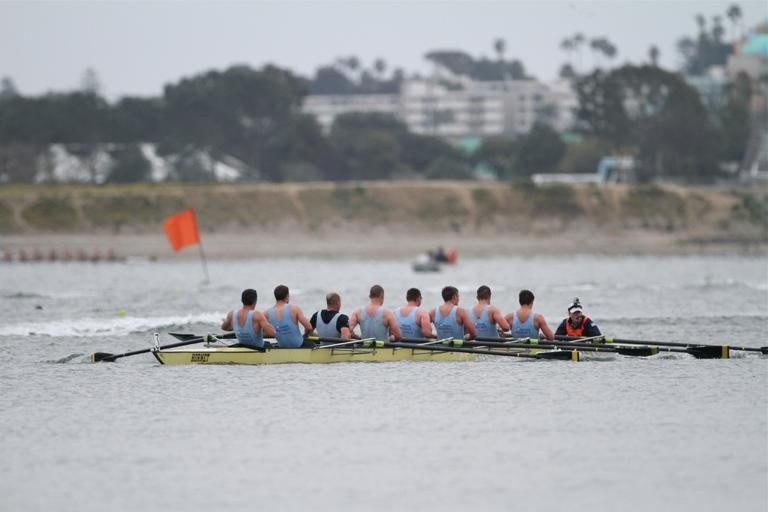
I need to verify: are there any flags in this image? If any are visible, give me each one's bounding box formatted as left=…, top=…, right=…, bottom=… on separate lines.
left=163, top=208, right=200, bottom=253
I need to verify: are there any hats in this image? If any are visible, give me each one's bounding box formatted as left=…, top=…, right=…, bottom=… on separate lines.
left=568, top=296, right=582, bottom=313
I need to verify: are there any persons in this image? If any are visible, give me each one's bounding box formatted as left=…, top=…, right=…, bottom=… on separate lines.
left=221, top=289, right=276, bottom=348
left=430, top=285, right=477, bottom=341
left=504, top=289, right=554, bottom=340
left=467, top=284, right=509, bottom=341
left=347, top=284, right=401, bottom=341
left=553, top=296, right=605, bottom=344
left=309, top=293, right=351, bottom=347
left=263, top=284, right=316, bottom=348
left=393, top=288, right=436, bottom=339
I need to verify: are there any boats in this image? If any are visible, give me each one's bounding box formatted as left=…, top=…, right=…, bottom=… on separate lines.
left=150, top=326, right=626, bottom=366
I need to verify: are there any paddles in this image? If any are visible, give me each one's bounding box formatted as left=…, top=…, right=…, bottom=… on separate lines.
left=169, top=334, right=235, bottom=340
left=305, top=337, right=583, bottom=362
left=390, top=338, right=659, bottom=356
left=93, top=333, right=236, bottom=362
left=511, top=339, right=729, bottom=359
